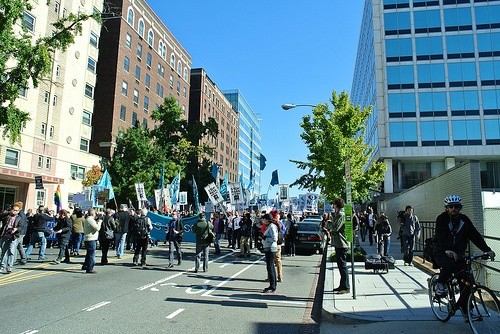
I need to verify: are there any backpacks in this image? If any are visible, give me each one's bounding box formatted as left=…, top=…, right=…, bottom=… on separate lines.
left=132, top=215, right=149, bottom=240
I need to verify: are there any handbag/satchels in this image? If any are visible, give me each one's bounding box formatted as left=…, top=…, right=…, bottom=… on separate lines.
left=105, top=227, right=117, bottom=240
left=203, top=221, right=216, bottom=243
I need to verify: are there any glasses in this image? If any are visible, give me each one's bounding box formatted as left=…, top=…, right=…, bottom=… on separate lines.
left=447, top=204, right=464, bottom=208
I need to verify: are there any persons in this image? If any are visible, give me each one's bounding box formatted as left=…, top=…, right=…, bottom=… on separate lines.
left=256, top=212, right=279, bottom=293
left=263, top=210, right=285, bottom=284
left=431, top=194, right=496, bottom=322
left=321, top=205, right=394, bottom=256
left=0, top=191, right=153, bottom=275
left=326, top=197, right=353, bottom=295
left=192, top=212, right=214, bottom=273
left=208, top=210, right=307, bottom=258
left=165, top=211, right=184, bottom=269
left=399, top=206, right=421, bottom=265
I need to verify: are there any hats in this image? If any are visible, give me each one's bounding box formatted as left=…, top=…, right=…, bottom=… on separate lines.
left=262, top=213, right=273, bottom=220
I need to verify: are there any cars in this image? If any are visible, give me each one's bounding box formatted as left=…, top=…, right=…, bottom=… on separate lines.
left=295, top=221, right=325, bottom=255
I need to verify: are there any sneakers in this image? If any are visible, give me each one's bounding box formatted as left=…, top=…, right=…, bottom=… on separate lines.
left=436, top=281, right=448, bottom=295
left=463, top=309, right=484, bottom=322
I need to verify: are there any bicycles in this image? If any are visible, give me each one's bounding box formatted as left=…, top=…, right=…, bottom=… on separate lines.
left=427, top=248, right=500, bottom=334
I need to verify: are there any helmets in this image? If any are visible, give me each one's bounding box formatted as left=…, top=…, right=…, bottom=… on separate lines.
left=443, top=195, right=467, bottom=206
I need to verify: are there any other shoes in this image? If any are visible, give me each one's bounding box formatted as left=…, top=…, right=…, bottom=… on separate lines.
left=177, top=259, right=183, bottom=266
left=0, top=241, right=157, bottom=274
left=165, top=263, right=175, bottom=268
left=333, top=286, right=351, bottom=294
left=195, top=265, right=209, bottom=274
left=404, top=261, right=417, bottom=266
left=209, top=238, right=297, bottom=258
left=265, top=277, right=284, bottom=282
left=262, top=287, right=278, bottom=293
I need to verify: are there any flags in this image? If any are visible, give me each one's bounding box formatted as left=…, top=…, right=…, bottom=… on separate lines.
left=50, top=168, right=229, bottom=213
